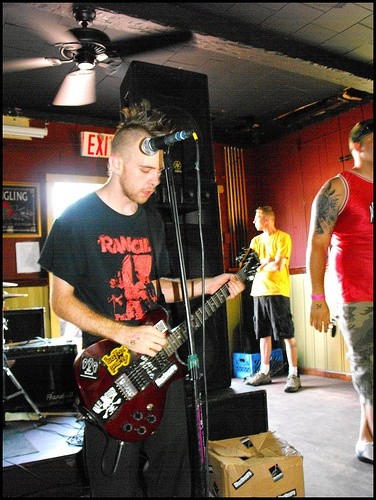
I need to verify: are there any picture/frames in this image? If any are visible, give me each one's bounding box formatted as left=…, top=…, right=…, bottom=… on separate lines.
left=3, top=181, right=42, bottom=238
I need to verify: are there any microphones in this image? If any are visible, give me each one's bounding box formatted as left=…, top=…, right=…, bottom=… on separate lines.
left=140, top=129, right=191, bottom=155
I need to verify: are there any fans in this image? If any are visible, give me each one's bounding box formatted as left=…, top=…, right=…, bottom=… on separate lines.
left=3, top=3, right=193, bottom=75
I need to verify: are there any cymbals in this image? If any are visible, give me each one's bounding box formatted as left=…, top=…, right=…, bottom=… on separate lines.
left=2, top=291, right=29, bottom=300
left=2, top=281, right=18, bottom=289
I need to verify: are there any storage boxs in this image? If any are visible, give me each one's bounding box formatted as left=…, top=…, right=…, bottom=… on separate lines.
left=207, top=431, right=305, bottom=497
left=233, top=348, right=284, bottom=378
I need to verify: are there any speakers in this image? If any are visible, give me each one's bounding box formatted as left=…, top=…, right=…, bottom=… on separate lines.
left=161, top=210, right=232, bottom=398
left=118, top=59, right=220, bottom=212
left=3, top=344, right=78, bottom=413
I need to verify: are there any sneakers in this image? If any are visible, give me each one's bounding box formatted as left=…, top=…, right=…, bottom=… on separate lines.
left=244, top=370, right=271, bottom=386
left=283, top=374, right=300, bottom=393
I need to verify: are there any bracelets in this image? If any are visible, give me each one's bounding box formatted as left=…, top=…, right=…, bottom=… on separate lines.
left=312, top=294, right=325, bottom=300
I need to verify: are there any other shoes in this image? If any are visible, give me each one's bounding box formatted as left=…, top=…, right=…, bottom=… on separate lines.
left=355, top=438, right=373, bottom=464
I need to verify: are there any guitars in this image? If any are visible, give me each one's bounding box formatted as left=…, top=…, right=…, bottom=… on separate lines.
left=71, top=246, right=262, bottom=442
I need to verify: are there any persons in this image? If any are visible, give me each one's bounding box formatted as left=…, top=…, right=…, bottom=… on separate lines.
left=37, top=98, right=245, bottom=499
left=308, top=118, right=374, bottom=464
left=246, top=205, right=302, bottom=393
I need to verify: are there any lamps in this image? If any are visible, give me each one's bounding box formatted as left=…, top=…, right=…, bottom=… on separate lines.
left=3, top=115, right=48, bottom=139
left=51, top=47, right=97, bottom=105
